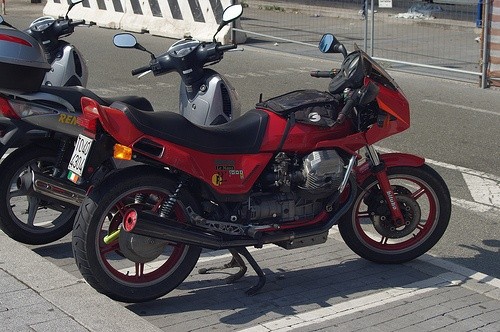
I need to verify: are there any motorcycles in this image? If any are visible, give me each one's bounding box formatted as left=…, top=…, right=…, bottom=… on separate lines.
left=67, top=33, right=452, bottom=303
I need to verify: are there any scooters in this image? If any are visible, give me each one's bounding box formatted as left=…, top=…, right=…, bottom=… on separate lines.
left=0, top=3, right=245, bottom=245
left=1, top=0, right=96, bottom=90
